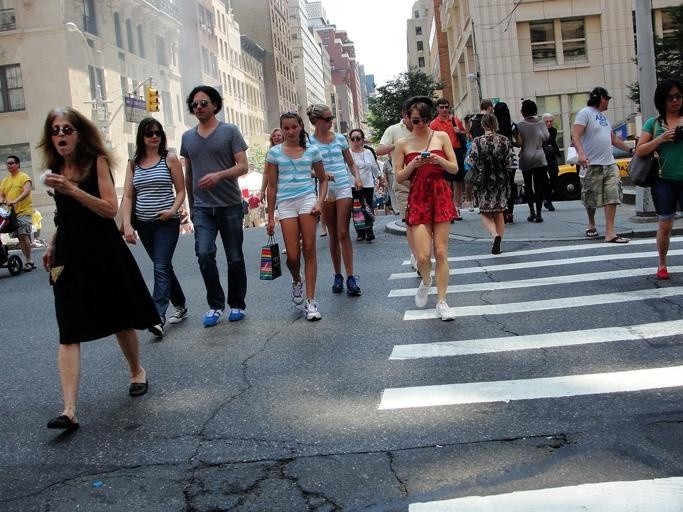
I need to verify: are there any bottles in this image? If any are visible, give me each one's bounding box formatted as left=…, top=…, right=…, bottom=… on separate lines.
left=351, top=199, right=362, bottom=207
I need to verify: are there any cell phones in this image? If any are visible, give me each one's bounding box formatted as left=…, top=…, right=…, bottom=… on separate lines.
left=421, top=152, right=430, bottom=158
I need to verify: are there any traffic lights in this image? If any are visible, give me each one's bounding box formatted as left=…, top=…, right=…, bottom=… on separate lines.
left=145, top=85, right=160, bottom=113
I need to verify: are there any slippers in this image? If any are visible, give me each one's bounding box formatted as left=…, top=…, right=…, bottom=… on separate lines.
left=46, top=415, right=80, bottom=430
left=585, top=228, right=630, bottom=243
left=129, top=379, right=149, bottom=396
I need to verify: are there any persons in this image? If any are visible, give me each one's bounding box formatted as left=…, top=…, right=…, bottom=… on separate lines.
left=178, top=85, right=249, bottom=328
left=120, top=115, right=186, bottom=337
left=0, top=155, right=36, bottom=272
left=32, top=210, right=43, bottom=241
left=35, top=104, right=162, bottom=430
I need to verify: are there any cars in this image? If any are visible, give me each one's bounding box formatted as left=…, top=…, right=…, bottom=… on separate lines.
left=547, top=137, right=640, bottom=200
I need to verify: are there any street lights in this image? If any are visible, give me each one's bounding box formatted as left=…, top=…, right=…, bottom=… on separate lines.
left=467, top=73, right=484, bottom=100
left=65, top=21, right=106, bottom=140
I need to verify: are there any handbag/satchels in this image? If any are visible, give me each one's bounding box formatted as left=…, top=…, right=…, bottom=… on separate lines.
left=113, top=187, right=137, bottom=235
left=258, top=242, right=282, bottom=281
left=566, top=146, right=580, bottom=165
left=625, top=148, right=659, bottom=187
left=48, top=209, right=65, bottom=289
left=351, top=186, right=378, bottom=232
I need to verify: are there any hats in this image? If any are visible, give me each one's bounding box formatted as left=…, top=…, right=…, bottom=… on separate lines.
left=590, top=87, right=611, bottom=99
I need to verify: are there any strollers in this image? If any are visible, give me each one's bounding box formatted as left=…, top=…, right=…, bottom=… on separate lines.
left=0, top=203, right=24, bottom=276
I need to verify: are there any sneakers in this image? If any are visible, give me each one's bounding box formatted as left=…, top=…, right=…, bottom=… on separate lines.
left=435, top=301, right=455, bottom=321
left=303, top=299, right=321, bottom=321
left=332, top=273, right=344, bottom=293
left=346, top=276, right=361, bottom=297
left=281, top=226, right=376, bottom=254
left=202, top=306, right=246, bottom=328
left=410, top=254, right=433, bottom=308
left=449, top=199, right=555, bottom=224
left=491, top=235, right=502, bottom=254
left=656, top=266, right=671, bottom=282
left=290, top=273, right=304, bottom=307
left=147, top=307, right=188, bottom=338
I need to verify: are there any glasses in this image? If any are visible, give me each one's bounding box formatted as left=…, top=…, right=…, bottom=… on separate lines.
left=317, top=116, right=334, bottom=122
left=143, top=129, right=161, bottom=138
left=190, top=100, right=212, bottom=108
left=665, top=93, right=683, bottom=101
left=351, top=137, right=363, bottom=142
left=49, top=127, right=79, bottom=136
left=411, top=118, right=430, bottom=125
left=438, top=106, right=449, bottom=109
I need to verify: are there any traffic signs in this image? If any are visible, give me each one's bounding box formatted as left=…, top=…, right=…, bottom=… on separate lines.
left=125, top=97, right=152, bottom=123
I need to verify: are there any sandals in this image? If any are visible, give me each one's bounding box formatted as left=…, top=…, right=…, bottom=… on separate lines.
left=22, top=261, right=37, bottom=272
